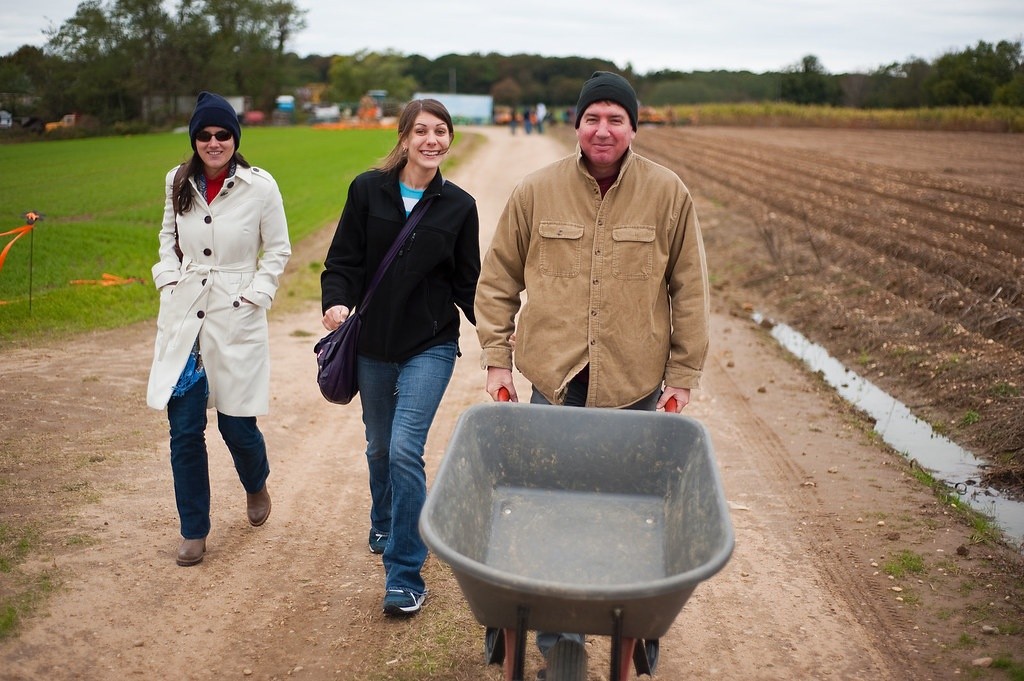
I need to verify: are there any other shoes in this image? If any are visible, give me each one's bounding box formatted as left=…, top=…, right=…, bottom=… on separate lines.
left=246, top=480, right=272, bottom=527
left=176, top=533, right=206, bottom=566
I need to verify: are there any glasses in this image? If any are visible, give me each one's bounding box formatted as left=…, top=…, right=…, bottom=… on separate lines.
left=194, top=130, right=233, bottom=142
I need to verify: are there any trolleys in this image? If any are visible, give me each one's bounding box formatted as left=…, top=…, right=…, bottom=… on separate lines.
left=417, top=387, right=736, bottom=680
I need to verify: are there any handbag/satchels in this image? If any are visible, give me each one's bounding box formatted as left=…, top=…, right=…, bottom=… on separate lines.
left=313, top=311, right=360, bottom=405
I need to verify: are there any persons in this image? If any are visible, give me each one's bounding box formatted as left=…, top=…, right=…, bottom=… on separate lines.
left=472, top=71, right=711, bottom=660
left=143, top=89, right=290, bottom=566
left=509, top=103, right=546, bottom=135
left=321, top=100, right=516, bottom=616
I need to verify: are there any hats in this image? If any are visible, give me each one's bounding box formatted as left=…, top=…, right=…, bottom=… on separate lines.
left=189, top=91, right=241, bottom=152
left=575, top=71, right=638, bottom=133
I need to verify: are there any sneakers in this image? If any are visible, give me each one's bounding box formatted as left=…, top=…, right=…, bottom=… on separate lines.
left=382, top=581, right=427, bottom=615
left=369, top=525, right=391, bottom=554
